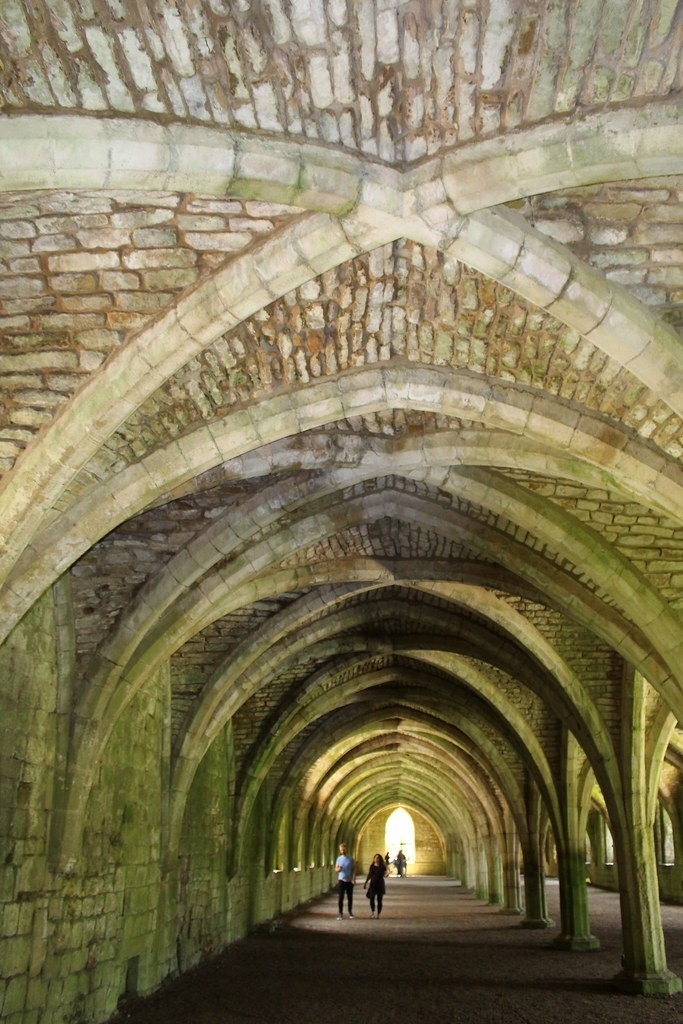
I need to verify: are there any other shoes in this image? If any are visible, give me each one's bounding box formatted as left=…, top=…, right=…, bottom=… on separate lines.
left=337, top=913, right=344, bottom=920
left=370, top=914, right=375, bottom=919
left=378, top=914, right=382, bottom=919
left=349, top=915, right=355, bottom=919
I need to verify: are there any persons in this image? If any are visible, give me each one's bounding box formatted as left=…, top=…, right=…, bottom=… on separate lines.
left=385, top=850, right=407, bottom=878
left=334, top=843, right=357, bottom=921
left=363, top=853, right=393, bottom=920
